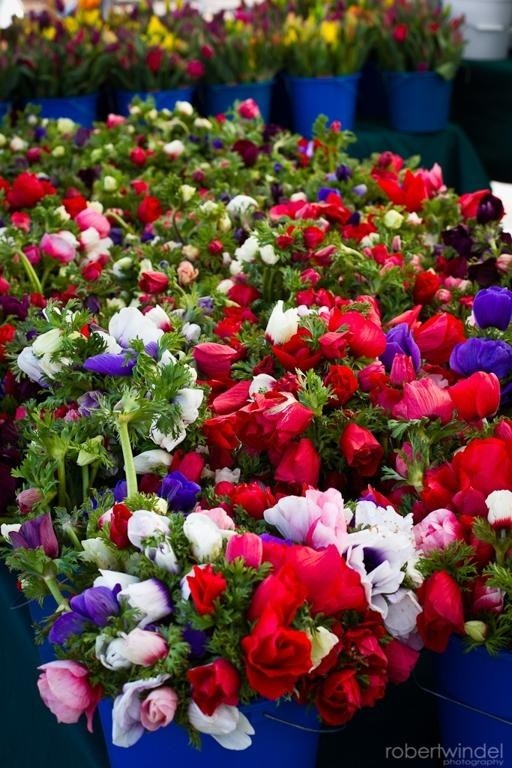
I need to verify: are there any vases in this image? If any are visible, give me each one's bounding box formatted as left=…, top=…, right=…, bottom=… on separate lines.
left=26, top=597, right=319, bottom=766
left=443, top=638, right=511, bottom=759
